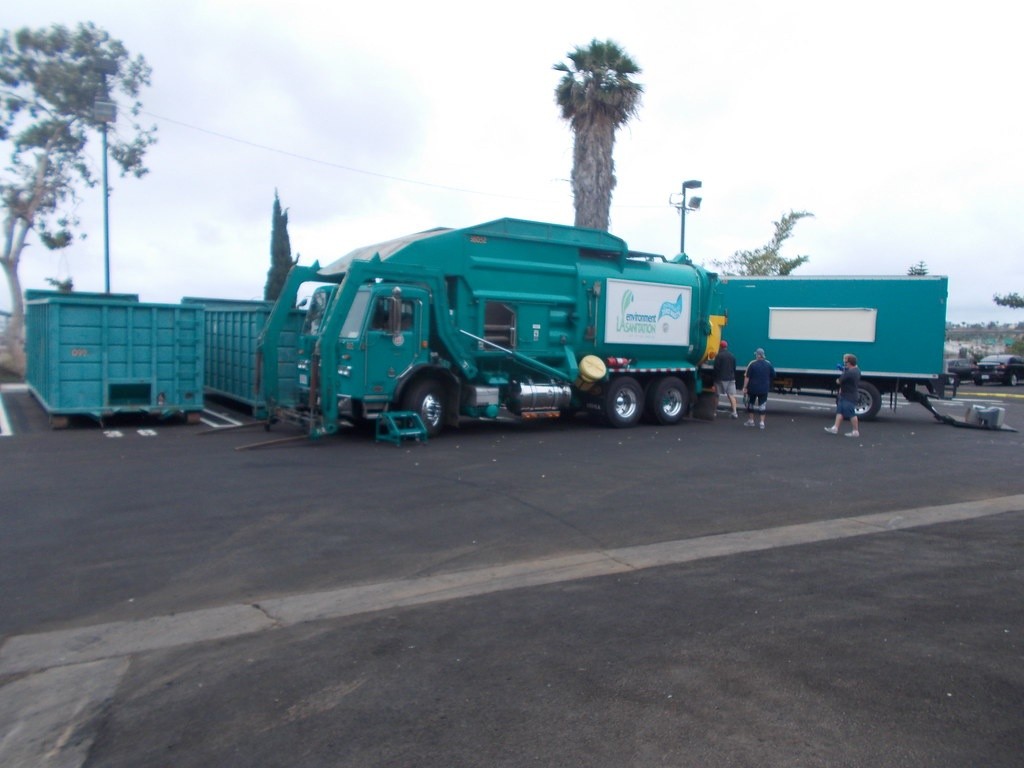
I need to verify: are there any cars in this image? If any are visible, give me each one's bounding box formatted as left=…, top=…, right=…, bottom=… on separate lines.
left=944, top=353, right=1024, bottom=386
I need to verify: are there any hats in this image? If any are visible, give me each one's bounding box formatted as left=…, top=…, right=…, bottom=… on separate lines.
left=754, top=348, right=764, bottom=356
left=720, top=340, right=727, bottom=347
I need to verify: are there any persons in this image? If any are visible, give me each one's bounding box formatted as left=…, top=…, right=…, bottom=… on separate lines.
left=823, top=354, right=861, bottom=437
left=743, top=348, right=775, bottom=429
left=712, top=340, right=739, bottom=419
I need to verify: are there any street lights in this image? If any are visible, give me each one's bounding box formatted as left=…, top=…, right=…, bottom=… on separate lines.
left=669, top=180, right=703, bottom=254
left=93, top=57, right=119, bottom=293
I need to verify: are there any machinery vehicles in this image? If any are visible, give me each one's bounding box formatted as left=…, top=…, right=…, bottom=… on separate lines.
left=195, top=216, right=728, bottom=451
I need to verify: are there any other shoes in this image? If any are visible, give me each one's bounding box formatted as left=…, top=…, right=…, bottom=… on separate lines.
left=744, top=419, right=756, bottom=427
left=730, top=412, right=739, bottom=419
left=844, top=430, right=860, bottom=437
left=759, top=422, right=766, bottom=430
left=824, top=426, right=839, bottom=435
